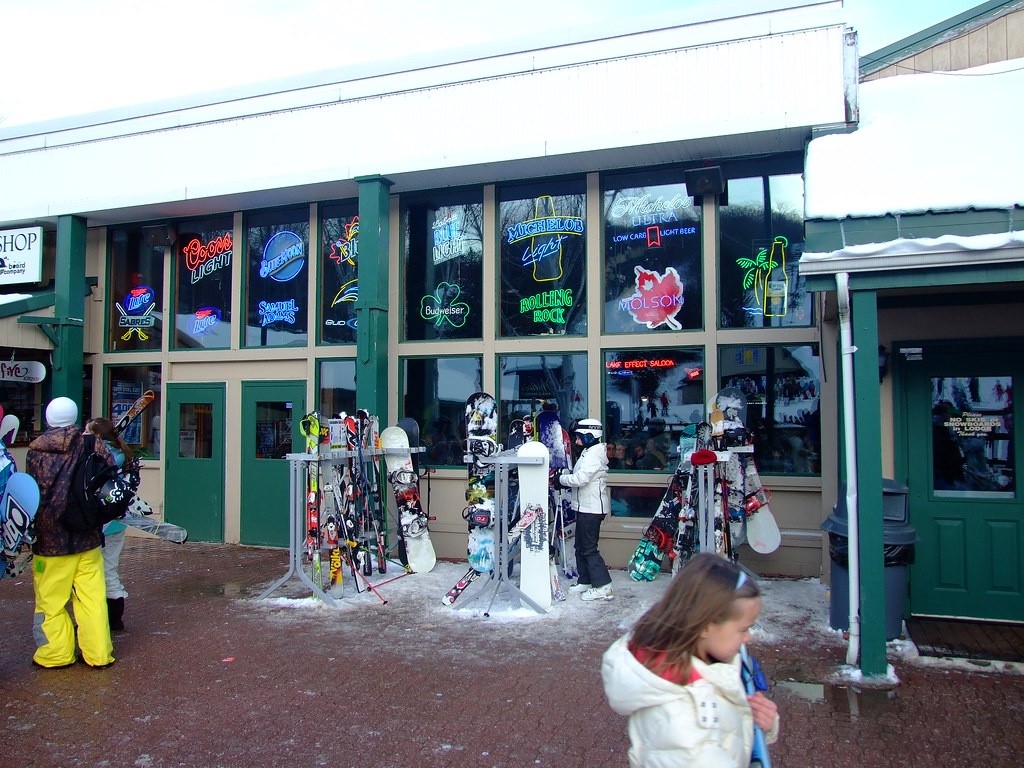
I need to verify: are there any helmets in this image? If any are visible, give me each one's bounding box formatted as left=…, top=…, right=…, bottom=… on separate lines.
left=649, top=419, right=665, bottom=430
left=575, top=418, right=602, bottom=438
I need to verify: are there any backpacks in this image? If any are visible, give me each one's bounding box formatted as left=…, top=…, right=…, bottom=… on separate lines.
left=62, top=435, right=126, bottom=530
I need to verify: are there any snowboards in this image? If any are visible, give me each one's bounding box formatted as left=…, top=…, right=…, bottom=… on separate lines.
left=0, top=471, right=41, bottom=582
left=490, top=409, right=578, bottom=579
left=378, top=425, right=436, bottom=573
left=462, top=392, right=498, bottom=573
left=394, top=416, right=421, bottom=570
left=625, top=387, right=782, bottom=581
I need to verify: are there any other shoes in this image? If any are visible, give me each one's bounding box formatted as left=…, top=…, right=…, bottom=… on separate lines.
left=107, top=598, right=125, bottom=631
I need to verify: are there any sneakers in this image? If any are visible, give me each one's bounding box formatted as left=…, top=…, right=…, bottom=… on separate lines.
left=582, top=584, right=614, bottom=601
left=569, top=584, right=591, bottom=590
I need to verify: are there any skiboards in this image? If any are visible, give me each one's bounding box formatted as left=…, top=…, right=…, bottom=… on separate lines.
left=107, top=390, right=189, bottom=544
left=295, top=406, right=380, bottom=601
left=441, top=504, right=567, bottom=606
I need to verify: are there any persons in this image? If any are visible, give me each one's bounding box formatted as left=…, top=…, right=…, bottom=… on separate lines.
left=82, top=418, right=144, bottom=632
left=551, top=418, right=615, bottom=601
left=605, top=433, right=680, bottom=517
left=932, top=376, right=1018, bottom=493
left=26, top=396, right=129, bottom=669
left=601, top=553, right=781, bottom=767
left=636, top=373, right=821, bottom=476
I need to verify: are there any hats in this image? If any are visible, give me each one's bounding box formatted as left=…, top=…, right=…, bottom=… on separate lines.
left=46, top=397, right=77, bottom=428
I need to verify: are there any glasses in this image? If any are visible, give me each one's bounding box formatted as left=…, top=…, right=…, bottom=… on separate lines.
left=608, top=447, right=615, bottom=450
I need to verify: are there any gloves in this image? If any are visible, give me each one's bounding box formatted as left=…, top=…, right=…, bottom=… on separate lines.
left=553, top=474, right=560, bottom=487
left=554, top=467, right=565, bottom=473
